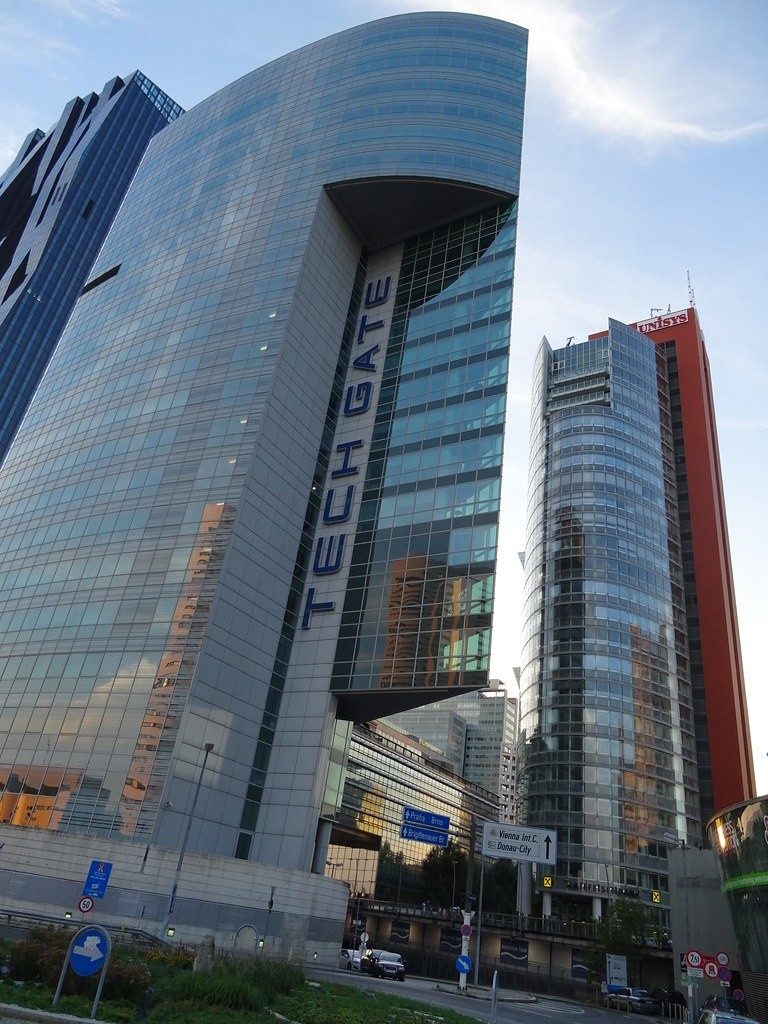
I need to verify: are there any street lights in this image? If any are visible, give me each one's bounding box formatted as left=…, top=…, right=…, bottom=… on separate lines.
left=160, top=742, right=217, bottom=940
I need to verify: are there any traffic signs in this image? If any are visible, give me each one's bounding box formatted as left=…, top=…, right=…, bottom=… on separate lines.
left=402, top=806, right=450, bottom=832
left=687, top=969, right=703, bottom=978
left=398, top=822, right=449, bottom=848
left=482, top=821, right=558, bottom=866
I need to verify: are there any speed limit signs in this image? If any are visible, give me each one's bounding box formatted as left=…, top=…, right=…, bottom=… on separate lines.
left=78, top=896, right=95, bottom=913
left=686, top=950, right=702, bottom=967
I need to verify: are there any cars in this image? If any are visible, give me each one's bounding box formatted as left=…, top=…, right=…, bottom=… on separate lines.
left=340, top=949, right=363, bottom=972
left=696, top=1008, right=760, bottom=1024
left=360, top=948, right=385, bottom=974
left=371, top=951, right=406, bottom=981
left=649, top=988, right=689, bottom=1016
left=701, top=994, right=759, bottom=1020
left=603, top=987, right=660, bottom=1014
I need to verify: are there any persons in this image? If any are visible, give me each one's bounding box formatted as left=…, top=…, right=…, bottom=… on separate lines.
left=550, top=914, right=669, bottom=946
left=419, top=899, right=462, bottom=919
